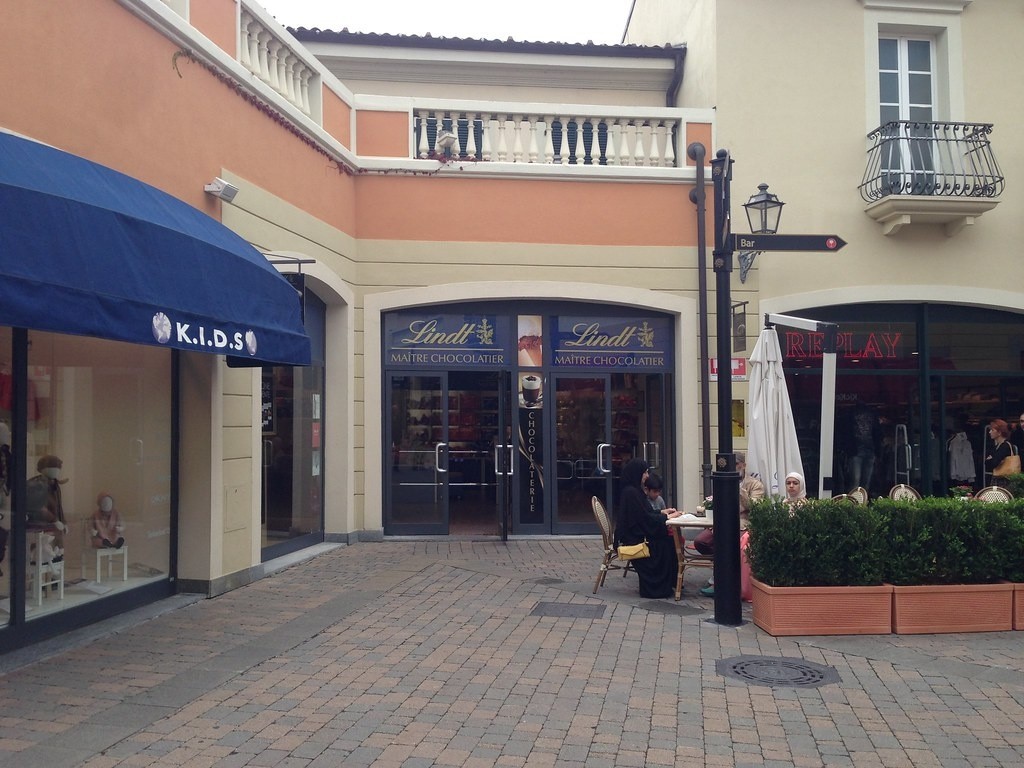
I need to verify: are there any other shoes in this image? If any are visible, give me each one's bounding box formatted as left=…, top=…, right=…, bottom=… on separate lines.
left=701, top=586, right=715, bottom=594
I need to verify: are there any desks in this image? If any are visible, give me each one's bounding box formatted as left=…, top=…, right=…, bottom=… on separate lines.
left=666, top=510, right=713, bottom=601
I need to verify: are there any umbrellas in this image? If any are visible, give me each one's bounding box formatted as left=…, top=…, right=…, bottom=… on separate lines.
left=747, top=314, right=806, bottom=503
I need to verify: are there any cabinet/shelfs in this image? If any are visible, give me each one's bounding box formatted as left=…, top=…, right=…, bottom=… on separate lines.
left=391, top=388, right=510, bottom=471
left=557, top=391, right=640, bottom=459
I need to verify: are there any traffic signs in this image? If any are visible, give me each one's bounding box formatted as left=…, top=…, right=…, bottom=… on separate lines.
left=733, top=232, right=848, bottom=253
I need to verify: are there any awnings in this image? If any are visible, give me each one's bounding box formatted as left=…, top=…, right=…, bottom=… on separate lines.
left=0, top=128, right=312, bottom=368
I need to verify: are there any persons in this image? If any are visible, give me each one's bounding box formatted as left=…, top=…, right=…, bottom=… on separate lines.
left=984, top=419, right=1014, bottom=494
left=391, top=404, right=402, bottom=472
left=699, top=472, right=808, bottom=602
left=614, top=457, right=679, bottom=598
left=694, top=451, right=764, bottom=554
left=88, top=492, right=125, bottom=549
left=1010, top=413, right=1024, bottom=474
left=26, top=455, right=68, bottom=590
left=846, top=393, right=884, bottom=491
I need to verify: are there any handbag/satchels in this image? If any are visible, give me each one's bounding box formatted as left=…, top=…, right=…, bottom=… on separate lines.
left=617, top=542, right=650, bottom=560
left=992, top=441, right=1021, bottom=477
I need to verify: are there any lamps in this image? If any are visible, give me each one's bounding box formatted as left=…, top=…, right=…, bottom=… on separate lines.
left=737, top=183, right=785, bottom=283
left=436, top=130, right=457, bottom=158
left=204, top=176, right=238, bottom=205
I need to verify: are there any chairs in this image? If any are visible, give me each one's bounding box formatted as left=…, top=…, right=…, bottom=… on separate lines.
left=888, top=484, right=921, bottom=502
left=976, top=486, right=1014, bottom=504
left=591, top=496, right=639, bottom=595
left=20, top=519, right=65, bottom=608
left=80, top=518, right=128, bottom=585
left=830, top=487, right=868, bottom=506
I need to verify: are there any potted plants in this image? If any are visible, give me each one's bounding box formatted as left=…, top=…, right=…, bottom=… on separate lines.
left=742, top=493, right=1024, bottom=637
